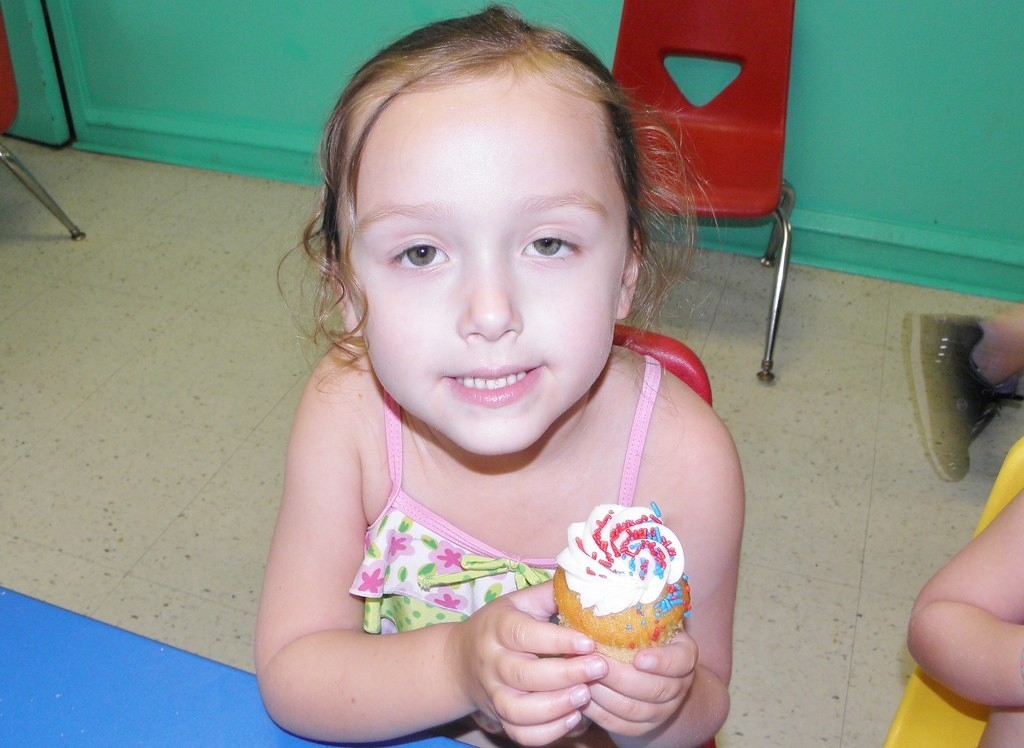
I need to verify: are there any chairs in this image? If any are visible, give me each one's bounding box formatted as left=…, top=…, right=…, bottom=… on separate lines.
left=0, top=11, right=88, bottom=240
left=607, top=0, right=796, bottom=384
left=881, top=438, right=1024, bottom=748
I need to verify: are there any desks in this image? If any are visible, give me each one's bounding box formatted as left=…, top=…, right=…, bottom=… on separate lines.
left=1, top=586, right=481, bottom=746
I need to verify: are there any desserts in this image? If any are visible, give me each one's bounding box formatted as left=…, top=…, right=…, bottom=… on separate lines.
left=554, top=503, right=690, bottom=662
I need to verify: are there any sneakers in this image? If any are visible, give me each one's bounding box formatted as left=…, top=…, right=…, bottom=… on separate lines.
left=905, top=312, right=1024, bottom=483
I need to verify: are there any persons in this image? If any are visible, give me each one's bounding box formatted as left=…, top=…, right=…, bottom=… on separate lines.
left=905, top=308, right=1024, bottom=480
left=254, top=4, right=745, bottom=748
left=907, top=488, right=1024, bottom=748
